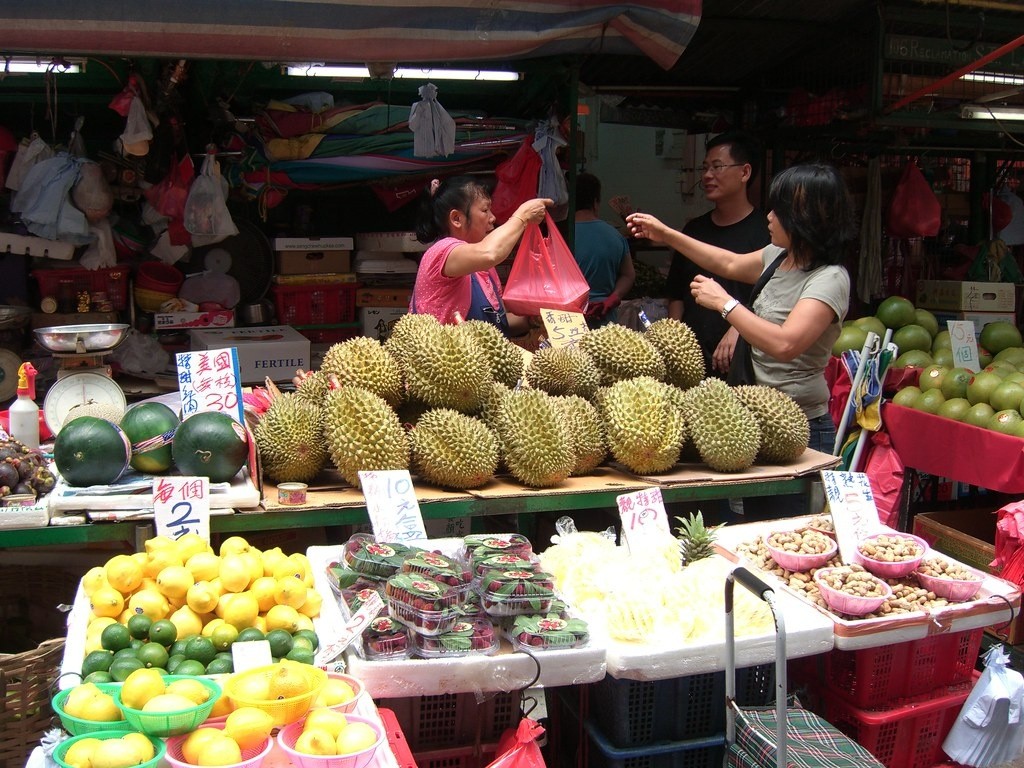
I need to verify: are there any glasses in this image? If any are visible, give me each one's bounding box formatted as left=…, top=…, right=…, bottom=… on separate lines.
left=700, top=162, right=748, bottom=175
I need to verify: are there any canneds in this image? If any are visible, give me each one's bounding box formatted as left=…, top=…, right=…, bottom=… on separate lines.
left=277, top=482, right=308, bottom=505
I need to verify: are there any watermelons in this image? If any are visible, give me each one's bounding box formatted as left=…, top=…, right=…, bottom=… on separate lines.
left=54, top=416, right=126, bottom=487
left=120, top=402, right=179, bottom=473
left=172, top=412, right=250, bottom=484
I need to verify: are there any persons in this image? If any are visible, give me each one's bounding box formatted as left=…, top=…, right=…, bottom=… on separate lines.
left=626, top=162, right=856, bottom=456
left=663, top=131, right=771, bottom=388
left=409, top=172, right=554, bottom=341
left=573, top=174, right=635, bottom=330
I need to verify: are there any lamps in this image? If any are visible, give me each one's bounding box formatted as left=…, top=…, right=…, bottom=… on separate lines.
left=958, top=70, right=1024, bottom=86
left=280, top=63, right=525, bottom=82
left=960, top=106, right=1024, bottom=120
left=0, top=55, right=88, bottom=74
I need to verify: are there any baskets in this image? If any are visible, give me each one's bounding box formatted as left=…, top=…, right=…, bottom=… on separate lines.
left=0, top=636, right=67, bottom=768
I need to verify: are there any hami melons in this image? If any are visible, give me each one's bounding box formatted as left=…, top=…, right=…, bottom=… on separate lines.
left=61, top=400, right=124, bottom=425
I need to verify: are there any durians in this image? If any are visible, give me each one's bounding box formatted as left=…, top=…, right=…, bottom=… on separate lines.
left=257, top=310, right=809, bottom=488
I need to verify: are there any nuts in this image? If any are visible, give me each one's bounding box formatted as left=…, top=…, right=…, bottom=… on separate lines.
left=735, top=521, right=980, bottom=622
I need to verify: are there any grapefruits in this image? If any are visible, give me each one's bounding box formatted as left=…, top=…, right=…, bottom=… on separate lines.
left=833, top=298, right=1024, bottom=437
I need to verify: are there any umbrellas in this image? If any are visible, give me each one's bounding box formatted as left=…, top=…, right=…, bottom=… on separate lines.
left=856, top=158, right=885, bottom=304
left=221, top=101, right=560, bottom=219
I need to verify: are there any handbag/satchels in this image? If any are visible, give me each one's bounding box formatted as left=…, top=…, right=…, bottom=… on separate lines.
left=726, top=332, right=757, bottom=387
left=501, top=210, right=590, bottom=316
left=887, top=162, right=941, bottom=236
left=182, top=152, right=240, bottom=247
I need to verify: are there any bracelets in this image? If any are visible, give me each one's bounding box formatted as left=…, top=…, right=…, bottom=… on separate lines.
left=528, top=315, right=541, bottom=329
left=510, top=215, right=526, bottom=229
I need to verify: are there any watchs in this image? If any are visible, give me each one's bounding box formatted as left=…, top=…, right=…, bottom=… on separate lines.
left=722, top=300, right=739, bottom=318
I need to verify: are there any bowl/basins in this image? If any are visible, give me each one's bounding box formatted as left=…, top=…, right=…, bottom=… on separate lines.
left=325, top=531, right=589, bottom=658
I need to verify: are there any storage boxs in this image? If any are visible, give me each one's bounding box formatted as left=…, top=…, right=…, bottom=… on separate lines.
left=915, top=279, right=1016, bottom=312
left=1, top=227, right=438, bottom=385
left=931, top=310, right=1016, bottom=345
left=28, top=497, right=1024, bottom=768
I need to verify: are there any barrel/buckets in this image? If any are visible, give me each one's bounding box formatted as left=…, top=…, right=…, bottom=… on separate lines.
left=244, top=304, right=269, bottom=323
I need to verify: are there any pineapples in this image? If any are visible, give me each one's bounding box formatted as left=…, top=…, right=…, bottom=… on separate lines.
left=545, top=510, right=773, bottom=646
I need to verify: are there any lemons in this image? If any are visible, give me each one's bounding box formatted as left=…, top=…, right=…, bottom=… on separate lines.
left=83, top=535, right=321, bottom=685
left=64, top=668, right=377, bottom=767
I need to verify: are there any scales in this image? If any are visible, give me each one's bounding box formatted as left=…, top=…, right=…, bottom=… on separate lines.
left=35, top=323, right=133, bottom=435
